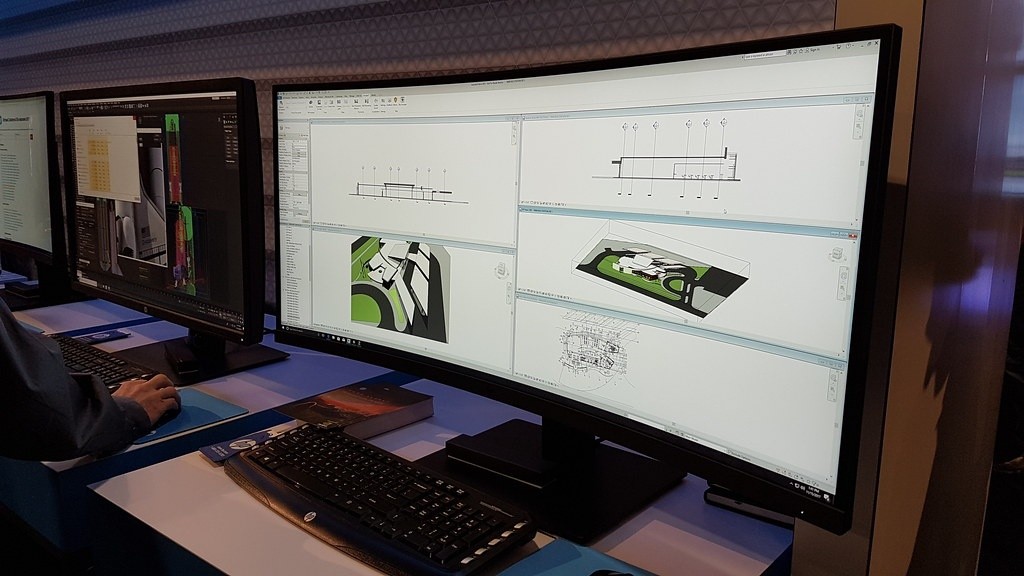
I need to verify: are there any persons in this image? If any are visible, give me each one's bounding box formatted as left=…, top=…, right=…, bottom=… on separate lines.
left=0, top=296, right=182, bottom=576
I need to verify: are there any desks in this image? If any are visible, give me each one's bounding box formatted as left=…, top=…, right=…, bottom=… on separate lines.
left=0, top=268, right=796, bottom=576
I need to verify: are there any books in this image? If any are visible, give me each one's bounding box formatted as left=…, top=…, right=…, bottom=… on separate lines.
left=283, top=382, right=435, bottom=440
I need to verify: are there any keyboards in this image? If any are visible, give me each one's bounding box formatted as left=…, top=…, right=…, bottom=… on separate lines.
left=47, top=332, right=156, bottom=395
left=224, top=423, right=536, bottom=576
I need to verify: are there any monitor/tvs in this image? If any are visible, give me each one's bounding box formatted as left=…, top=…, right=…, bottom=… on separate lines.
left=60, top=76, right=291, bottom=388
left=0, top=90, right=100, bottom=312
left=275, top=24, right=903, bottom=549
